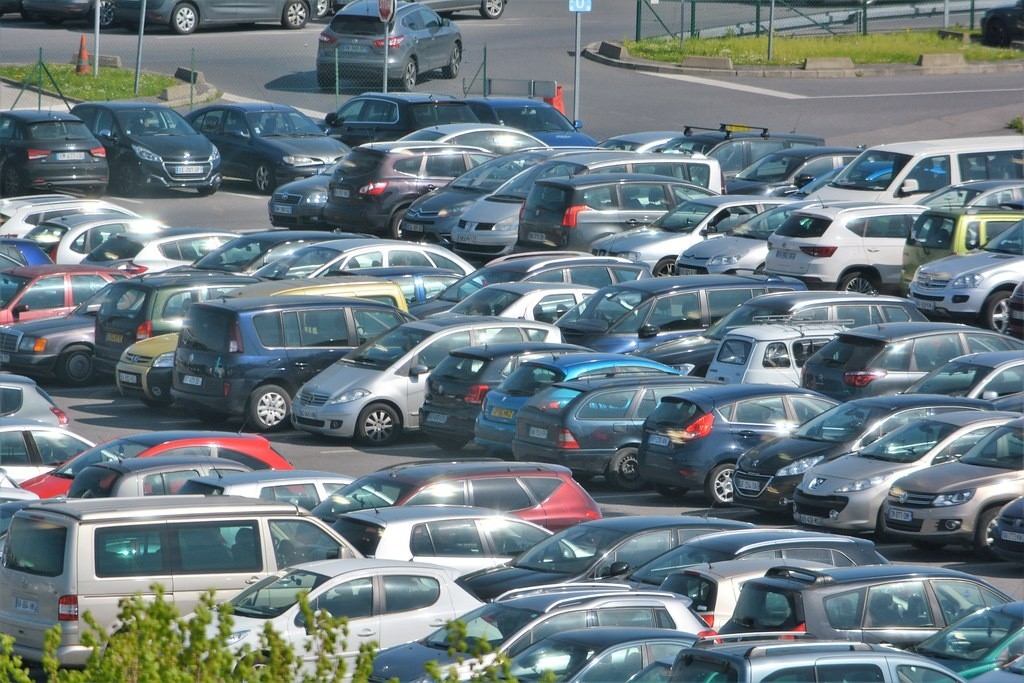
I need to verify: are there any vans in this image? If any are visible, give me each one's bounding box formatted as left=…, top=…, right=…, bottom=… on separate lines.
left=808, top=134, right=1024, bottom=201
left=0, top=492, right=365, bottom=670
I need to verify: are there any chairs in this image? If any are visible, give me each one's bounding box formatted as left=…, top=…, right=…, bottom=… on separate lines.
left=793, top=343, right=803, bottom=367
left=534, top=303, right=569, bottom=323
left=885, top=218, right=905, bottom=236
left=182, top=298, right=191, bottom=311
left=263, top=118, right=276, bottom=134
left=651, top=298, right=700, bottom=331
left=917, top=158, right=947, bottom=190
left=621, top=187, right=668, bottom=210
left=934, top=342, right=958, bottom=365
left=370, top=103, right=389, bottom=121
left=231, top=527, right=256, bottom=568
left=304, top=313, right=318, bottom=343
left=902, top=595, right=928, bottom=625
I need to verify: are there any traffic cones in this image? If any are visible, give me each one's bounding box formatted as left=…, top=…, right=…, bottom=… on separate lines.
left=75, top=34, right=93, bottom=75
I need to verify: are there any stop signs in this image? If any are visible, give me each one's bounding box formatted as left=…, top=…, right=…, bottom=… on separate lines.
left=378, top=0, right=393, bottom=22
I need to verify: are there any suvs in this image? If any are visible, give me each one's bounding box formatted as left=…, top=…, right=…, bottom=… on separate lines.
left=368, top=580, right=723, bottom=683
left=1, top=191, right=655, bottom=447
left=305, top=456, right=605, bottom=535
left=553, top=272, right=1024, bottom=419
left=670, top=563, right=1024, bottom=683
left=459, top=511, right=889, bottom=590
left=317, top=0, right=462, bottom=92
left=981, top=0, right=1024, bottom=49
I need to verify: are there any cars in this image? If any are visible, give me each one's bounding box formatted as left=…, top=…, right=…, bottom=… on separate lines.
left=0, top=0, right=330, bottom=36
left=263, top=121, right=1024, bottom=335
left=173, top=556, right=488, bottom=682
left=321, top=0, right=508, bottom=21
left=419, top=341, right=1024, bottom=571
left=333, top=502, right=598, bottom=579
left=78, top=90, right=602, bottom=196
left=0, top=367, right=397, bottom=556
